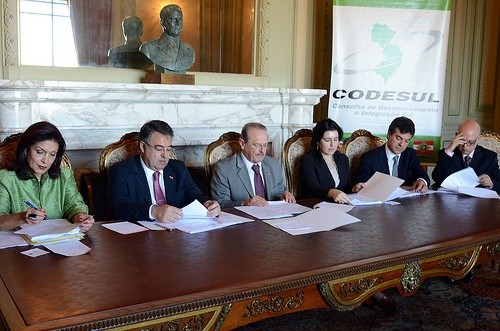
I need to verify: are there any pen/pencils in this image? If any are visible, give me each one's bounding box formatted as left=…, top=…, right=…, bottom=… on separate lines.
left=154, top=224, right=172, bottom=232
left=23, top=199, right=50, bottom=220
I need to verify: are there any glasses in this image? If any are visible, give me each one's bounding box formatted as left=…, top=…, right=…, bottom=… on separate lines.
left=460, top=137, right=477, bottom=146
left=143, top=141, right=175, bottom=153
left=241, top=137, right=270, bottom=149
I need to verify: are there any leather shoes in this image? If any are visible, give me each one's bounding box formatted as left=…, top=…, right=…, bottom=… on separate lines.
left=378, top=294, right=400, bottom=311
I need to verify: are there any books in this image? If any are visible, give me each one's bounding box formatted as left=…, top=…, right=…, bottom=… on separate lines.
left=0, top=167, right=500, bottom=257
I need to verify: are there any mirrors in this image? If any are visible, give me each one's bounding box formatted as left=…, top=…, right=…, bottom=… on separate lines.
left=0, top=0, right=271, bottom=88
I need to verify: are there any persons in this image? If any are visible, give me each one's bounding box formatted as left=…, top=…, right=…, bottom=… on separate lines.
left=352, top=117, right=430, bottom=193
left=107, top=120, right=221, bottom=222
left=0, top=121, right=94, bottom=232
left=139, top=4, right=195, bottom=73
left=431, top=120, right=500, bottom=194
left=297, top=119, right=366, bottom=203
left=108, top=16, right=153, bottom=69
left=211, top=123, right=296, bottom=206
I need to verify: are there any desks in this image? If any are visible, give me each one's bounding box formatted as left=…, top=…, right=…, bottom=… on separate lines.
left=0, top=188, right=500, bottom=331
left=73, top=162, right=205, bottom=215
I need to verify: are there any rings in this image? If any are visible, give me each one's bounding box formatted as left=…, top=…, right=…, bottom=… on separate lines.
left=28, top=214, right=36, bottom=221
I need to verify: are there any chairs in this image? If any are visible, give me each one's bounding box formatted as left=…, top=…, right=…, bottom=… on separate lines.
left=477, top=130, right=500, bottom=170
left=99, top=132, right=141, bottom=222
left=283, top=129, right=313, bottom=199
left=343, top=130, right=384, bottom=184
left=0, top=133, right=71, bottom=173
left=205, top=132, right=241, bottom=196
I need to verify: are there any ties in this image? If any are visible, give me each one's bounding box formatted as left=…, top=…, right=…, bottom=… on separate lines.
left=465, top=156, right=471, bottom=166
left=252, top=165, right=265, bottom=199
left=392, top=155, right=399, bottom=178
left=153, top=171, right=166, bottom=206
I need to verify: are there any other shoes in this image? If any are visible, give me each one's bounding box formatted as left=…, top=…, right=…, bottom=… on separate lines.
left=462, top=269, right=474, bottom=284
left=441, top=277, right=457, bottom=287
